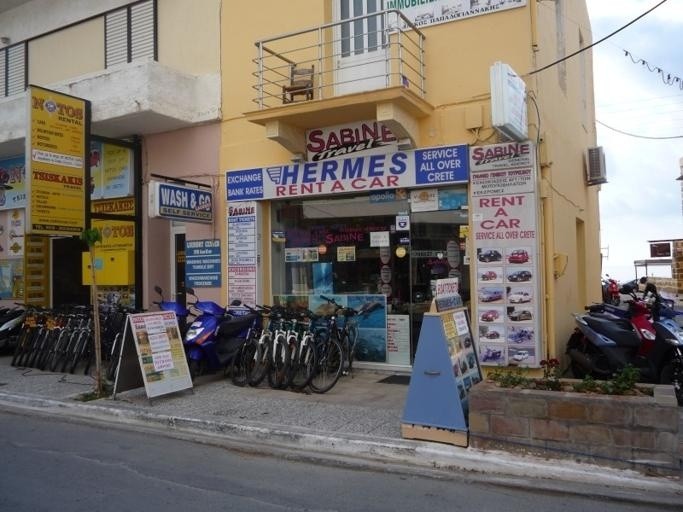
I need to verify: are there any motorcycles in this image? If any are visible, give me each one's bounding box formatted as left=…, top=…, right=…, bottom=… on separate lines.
left=566, top=271, right=683, bottom=407
left=150, top=285, right=198, bottom=343
left=0, top=306, right=24, bottom=353
left=180, top=284, right=262, bottom=376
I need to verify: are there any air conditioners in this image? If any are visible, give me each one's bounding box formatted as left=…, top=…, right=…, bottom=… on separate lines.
left=584, top=146, right=609, bottom=186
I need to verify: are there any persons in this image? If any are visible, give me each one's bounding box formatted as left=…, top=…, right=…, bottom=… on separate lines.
left=636, top=276, right=660, bottom=322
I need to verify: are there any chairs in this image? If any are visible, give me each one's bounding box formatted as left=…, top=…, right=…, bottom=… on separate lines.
left=282, top=64, right=315, bottom=104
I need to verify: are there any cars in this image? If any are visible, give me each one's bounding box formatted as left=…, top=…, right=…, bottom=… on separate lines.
left=449, top=338, right=479, bottom=400
left=478, top=248, right=532, bottom=363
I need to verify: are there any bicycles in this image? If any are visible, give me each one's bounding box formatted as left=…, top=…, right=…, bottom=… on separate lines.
left=10, top=301, right=149, bottom=381
left=228, top=294, right=360, bottom=393
